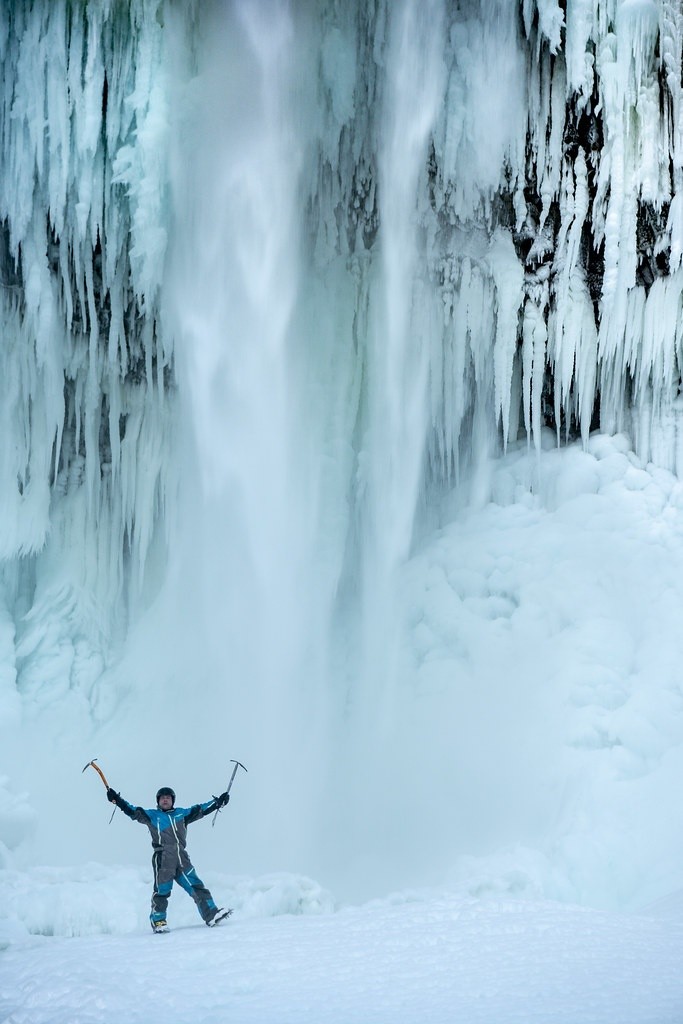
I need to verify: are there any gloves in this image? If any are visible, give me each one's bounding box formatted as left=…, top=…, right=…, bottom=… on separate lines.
left=219, top=792, right=229, bottom=805
left=107, top=789, right=119, bottom=804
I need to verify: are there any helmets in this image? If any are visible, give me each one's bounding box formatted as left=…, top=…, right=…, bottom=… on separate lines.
left=156, top=787, right=175, bottom=807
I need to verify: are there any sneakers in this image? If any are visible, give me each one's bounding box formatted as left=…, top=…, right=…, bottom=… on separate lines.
left=151, top=919, right=169, bottom=933
left=206, top=908, right=229, bottom=927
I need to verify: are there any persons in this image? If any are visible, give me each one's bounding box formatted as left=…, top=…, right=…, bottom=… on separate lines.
left=107, top=787, right=230, bottom=933
